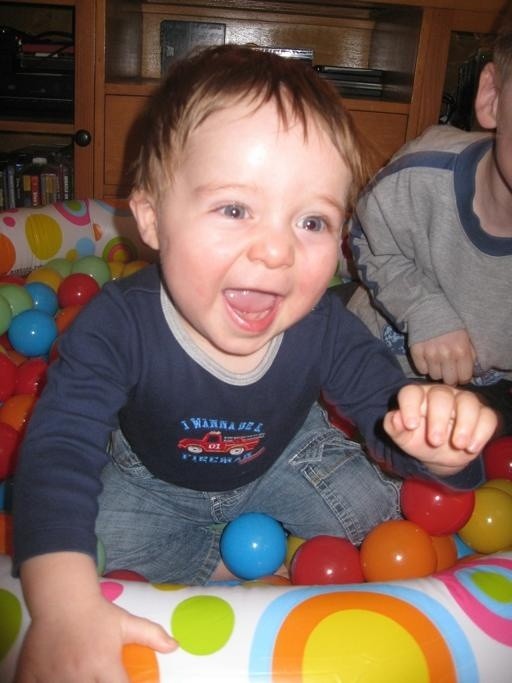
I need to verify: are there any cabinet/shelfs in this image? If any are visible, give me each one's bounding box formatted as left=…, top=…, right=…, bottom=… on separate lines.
left=1, top=0, right=512, bottom=202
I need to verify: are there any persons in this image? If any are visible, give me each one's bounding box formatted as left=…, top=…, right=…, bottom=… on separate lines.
left=7, top=39, right=500, bottom=683
left=335, top=2, right=512, bottom=465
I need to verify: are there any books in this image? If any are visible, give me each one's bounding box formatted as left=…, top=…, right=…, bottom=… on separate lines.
left=0, top=141, right=76, bottom=214
